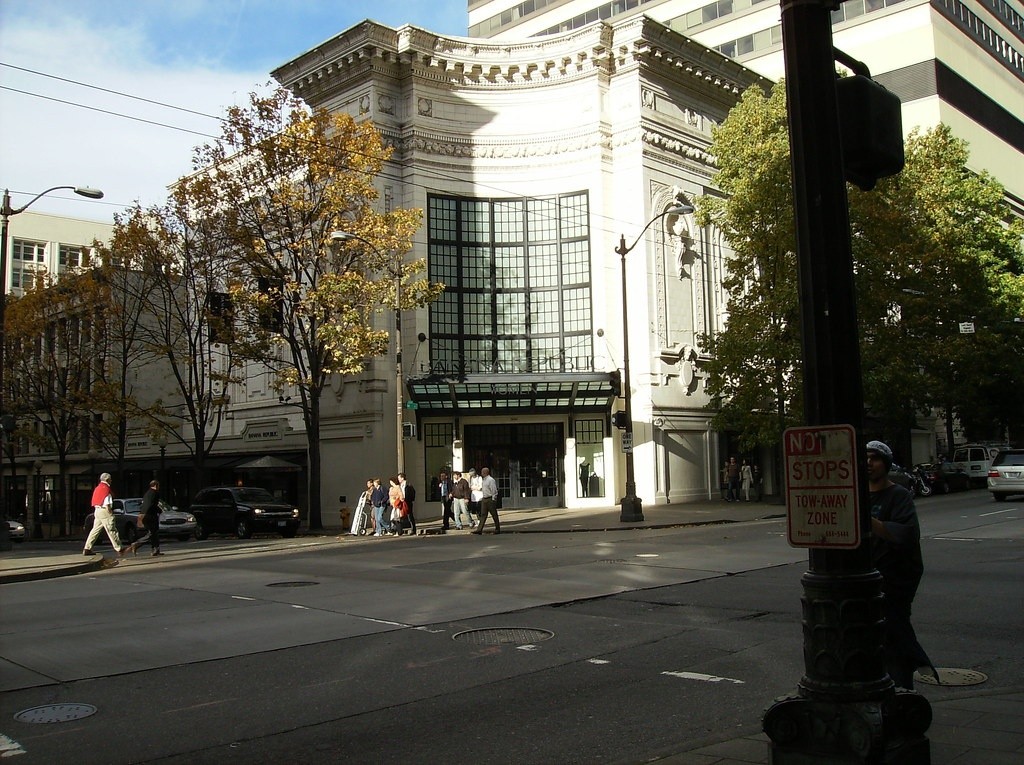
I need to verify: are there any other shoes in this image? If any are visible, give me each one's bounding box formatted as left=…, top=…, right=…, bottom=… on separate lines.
left=82, top=550, right=96, bottom=555
left=398, top=531, right=404, bottom=535
left=117, top=547, right=131, bottom=557
left=474, top=520, right=480, bottom=527
left=471, top=531, right=482, bottom=535
left=369, top=531, right=376, bottom=535
left=132, top=543, right=138, bottom=557
left=374, top=533, right=382, bottom=536
left=410, top=531, right=416, bottom=535
left=441, top=526, right=450, bottom=530
left=385, top=532, right=394, bottom=535
left=493, top=532, right=501, bottom=534
left=151, top=552, right=164, bottom=556
left=391, top=530, right=396, bottom=535
left=470, top=522, right=475, bottom=529
left=455, top=528, right=463, bottom=530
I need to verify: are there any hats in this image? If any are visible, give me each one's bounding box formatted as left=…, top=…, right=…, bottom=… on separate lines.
left=867, top=440, right=893, bottom=464
left=100, top=473, right=111, bottom=481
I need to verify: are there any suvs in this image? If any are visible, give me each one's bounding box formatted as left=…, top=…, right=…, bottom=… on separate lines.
left=187, top=485, right=301, bottom=538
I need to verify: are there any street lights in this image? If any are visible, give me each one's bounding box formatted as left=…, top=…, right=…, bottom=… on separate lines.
left=157, top=437, right=168, bottom=496
left=617, top=204, right=694, bottom=522
left=31, top=458, right=45, bottom=540
left=87, top=446, right=97, bottom=494
left=330, top=229, right=407, bottom=479
left=1, top=186, right=104, bottom=420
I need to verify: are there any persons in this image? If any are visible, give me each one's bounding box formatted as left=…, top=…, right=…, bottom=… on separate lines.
left=366, top=473, right=415, bottom=536
left=579, top=460, right=590, bottom=497
left=471, top=468, right=500, bottom=535
left=438, top=471, right=475, bottom=530
left=132, top=480, right=164, bottom=556
left=466, top=468, right=482, bottom=527
left=83, top=473, right=131, bottom=556
left=741, top=459, right=753, bottom=501
left=752, top=464, right=763, bottom=501
left=863, top=440, right=938, bottom=693
left=725, top=456, right=742, bottom=502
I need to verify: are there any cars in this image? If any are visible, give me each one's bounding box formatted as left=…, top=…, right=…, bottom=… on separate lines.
left=3, top=514, right=26, bottom=543
left=987, top=449, right=1024, bottom=502
left=82, top=497, right=198, bottom=543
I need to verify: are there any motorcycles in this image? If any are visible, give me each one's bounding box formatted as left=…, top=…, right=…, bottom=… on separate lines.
left=891, top=457, right=950, bottom=498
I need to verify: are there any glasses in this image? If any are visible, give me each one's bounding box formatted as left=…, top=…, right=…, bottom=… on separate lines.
left=867, top=454, right=880, bottom=461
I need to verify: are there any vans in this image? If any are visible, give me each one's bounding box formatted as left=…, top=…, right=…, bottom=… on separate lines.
left=951, top=445, right=1013, bottom=484
